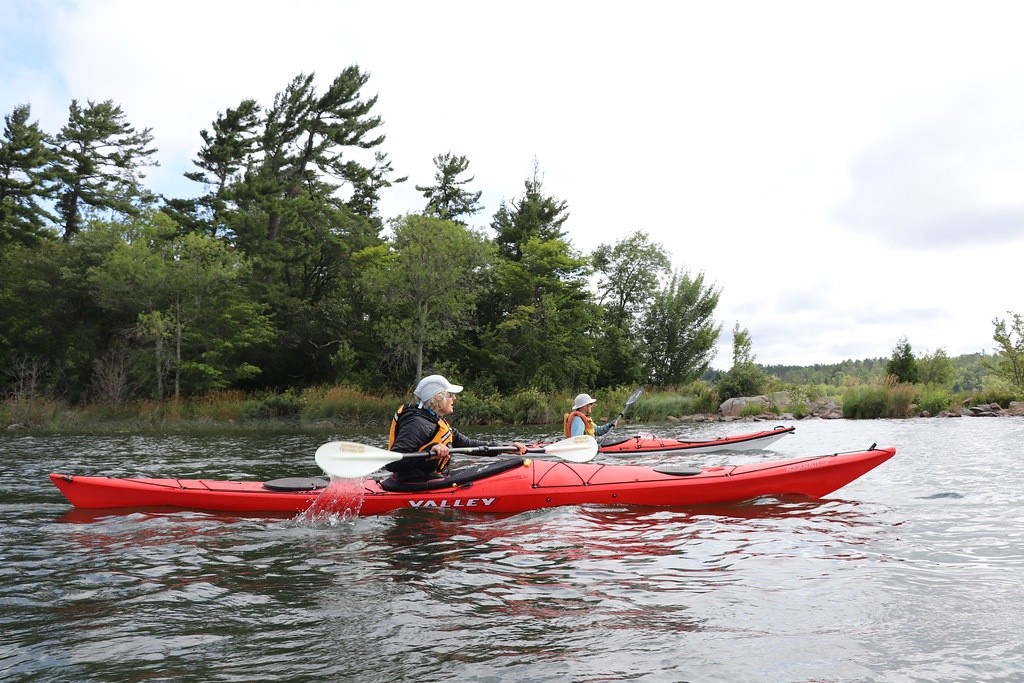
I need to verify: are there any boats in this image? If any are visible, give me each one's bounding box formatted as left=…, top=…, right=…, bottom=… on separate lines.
left=521, top=424, right=799, bottom=458
left=49, top=441, right=897, bottom=522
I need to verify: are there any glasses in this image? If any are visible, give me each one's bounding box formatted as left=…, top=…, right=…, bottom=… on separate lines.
left=445, top=392, right=452, bottom=398
left=588, top=403, right=592, bottom=405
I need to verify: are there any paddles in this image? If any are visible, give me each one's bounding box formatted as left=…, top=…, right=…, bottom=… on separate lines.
left=316, top=431, right=600, bottom=481
left=601, top=386, right=644, bottom=446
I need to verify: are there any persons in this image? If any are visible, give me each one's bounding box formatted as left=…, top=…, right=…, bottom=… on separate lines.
left=564, top=394, right=618, bottom=440
left=385, top=375, right=527, bottom=481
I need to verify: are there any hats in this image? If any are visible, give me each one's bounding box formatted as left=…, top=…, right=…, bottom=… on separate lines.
left=572, top=394, right=596, bottom=410
left=414, top=375, right=463, bottom=409
